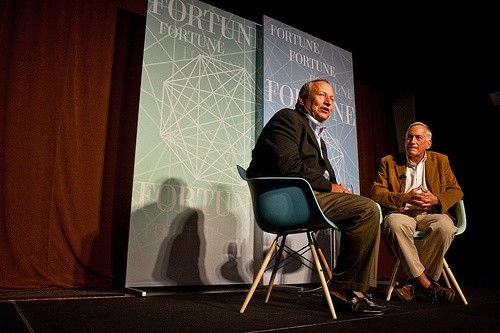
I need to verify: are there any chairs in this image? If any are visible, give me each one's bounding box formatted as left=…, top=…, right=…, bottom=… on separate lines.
left=233, top=165, right=384, bottom=320
left=375, top=193, right=468, bottom=305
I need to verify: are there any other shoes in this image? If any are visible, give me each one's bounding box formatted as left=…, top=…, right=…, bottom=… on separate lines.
left=426, top=281, right=455, bottom=306
left=394, top=281, right=415, bottom=302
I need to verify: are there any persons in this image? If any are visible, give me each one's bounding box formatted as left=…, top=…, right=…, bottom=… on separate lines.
left=246, top=79, right=390, bottom=316
left=371, top=122, right=464, bottom=304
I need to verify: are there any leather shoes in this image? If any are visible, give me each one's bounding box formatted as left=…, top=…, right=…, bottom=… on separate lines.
left=321, top=293, right=384, bottom=316
left=359, top=295, right=390, bottom=311
